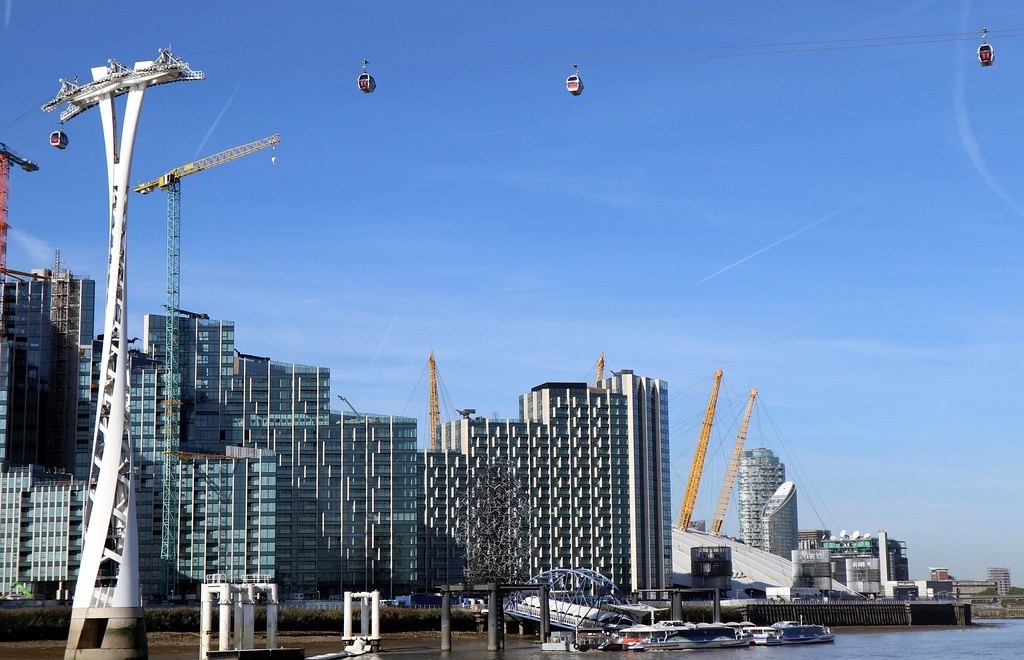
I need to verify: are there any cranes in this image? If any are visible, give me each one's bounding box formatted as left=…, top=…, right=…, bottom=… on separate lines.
left=131, top=131, right=281, bottom=194
left=0, top=142, right=40, bottom=172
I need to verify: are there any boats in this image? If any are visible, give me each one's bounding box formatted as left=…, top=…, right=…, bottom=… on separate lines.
left=569, top=614, right=836, bottom=654
